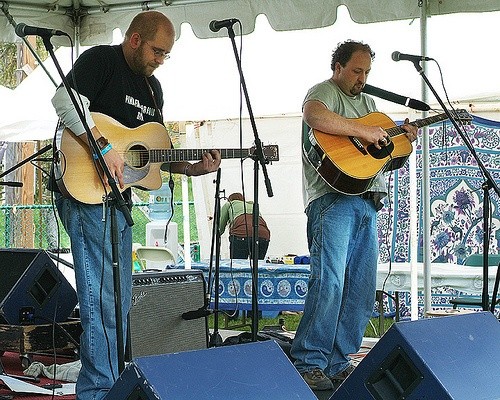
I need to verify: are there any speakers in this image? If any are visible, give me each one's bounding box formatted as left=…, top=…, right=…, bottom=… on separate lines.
left=127, top=269, right=208, bottom=360
left=0, top=246, right=79, bottom=323
left=102, top=338, right=319, bottom=400
left=328, top=310, right=500, bottom=400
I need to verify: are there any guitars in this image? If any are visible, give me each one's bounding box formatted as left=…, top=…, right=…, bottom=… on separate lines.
left=301, top=107, right=474, bottom=196
left=54, top=111, right=280, bottom=207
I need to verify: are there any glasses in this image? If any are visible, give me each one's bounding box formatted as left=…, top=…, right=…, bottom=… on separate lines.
left=143, top=41, right=170, bottom=61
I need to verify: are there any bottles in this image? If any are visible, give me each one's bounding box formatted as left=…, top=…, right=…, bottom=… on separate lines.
left=148, top=183, right=172, bottom=219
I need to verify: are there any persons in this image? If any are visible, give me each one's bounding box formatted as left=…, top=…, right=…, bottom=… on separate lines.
left=51, top=12, right=219, bottom=400
left=221, top=191, right=271, bottom=321
left=290, top=39, right=417, bottom=392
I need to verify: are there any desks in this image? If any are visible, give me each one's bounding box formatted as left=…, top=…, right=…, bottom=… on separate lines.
left=166, top=259, right=312, bottom=330
left=372, top=263, right=500, bottom=337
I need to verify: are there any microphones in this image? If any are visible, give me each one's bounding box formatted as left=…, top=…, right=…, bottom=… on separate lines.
left=15, top=22, right=67, bottom=37
left=182, top=308, right=221, bottom=320
left=209, top=18, right=239, bottom=32
left=392, top=51, right=434, bottom=62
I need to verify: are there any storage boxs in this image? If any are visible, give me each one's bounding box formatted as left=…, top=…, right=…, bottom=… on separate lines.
left=283, top=254, right=310, bottom=264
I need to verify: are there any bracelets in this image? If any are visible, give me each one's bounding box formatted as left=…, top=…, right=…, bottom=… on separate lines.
left=92, top=137, right=111, bottom=159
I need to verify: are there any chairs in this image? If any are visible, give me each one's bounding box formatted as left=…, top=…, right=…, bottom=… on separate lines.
left=426, top=255, right=499, bottom=309
left=137, top=246, right=176, bottom=273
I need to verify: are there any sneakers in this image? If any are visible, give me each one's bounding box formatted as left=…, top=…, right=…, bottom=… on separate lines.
left=301, top=369, right=333, bottom=390
left=332, top=366, right=355, bottom=382
